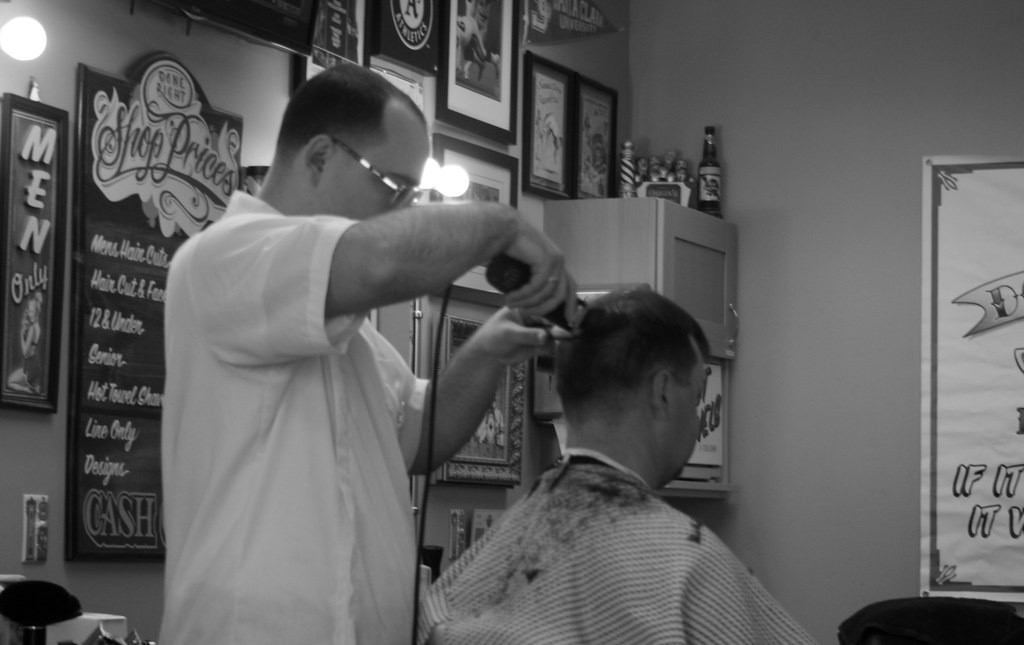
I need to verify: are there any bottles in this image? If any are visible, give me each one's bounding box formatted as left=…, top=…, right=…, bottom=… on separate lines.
left=698, top=124, right=722, bottom=213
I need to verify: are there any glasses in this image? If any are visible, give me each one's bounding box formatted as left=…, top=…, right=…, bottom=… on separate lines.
left=331, top=137, right=422, bottom=210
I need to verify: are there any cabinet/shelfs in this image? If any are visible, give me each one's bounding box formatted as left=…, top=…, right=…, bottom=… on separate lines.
left=535, top=182, right=742, bottom=495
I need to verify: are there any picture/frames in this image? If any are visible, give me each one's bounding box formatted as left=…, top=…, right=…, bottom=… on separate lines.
left=573, top=73, right=620, bottom=202
left=427, top=279, right=523, bottom=489
left=520, top=48, right=575, bottom=202
left=430, top=134, right=520, bottom=309
left=437, top=0, right=520, bottom=145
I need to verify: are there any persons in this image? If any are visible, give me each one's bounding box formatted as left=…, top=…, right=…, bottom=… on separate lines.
left=416, top=286, right=824, bottom=645
left=157, top=62, right=593, bottom=643
